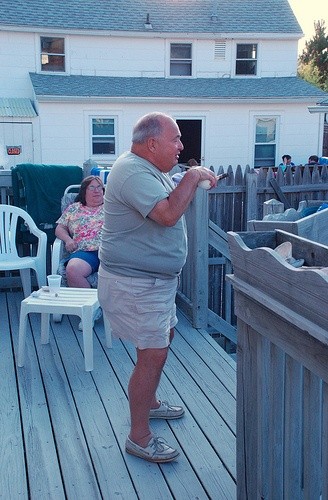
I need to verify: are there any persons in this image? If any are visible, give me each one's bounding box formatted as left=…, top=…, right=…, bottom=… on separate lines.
left=98, top=112, right=218, bottom=462
left=185, top=159, right=199, bottom=166
left=278, top=155, right=296, bottom=172
left=55, top=175, right=105, bottom=330
left=301, top=155, right=322, bottom=178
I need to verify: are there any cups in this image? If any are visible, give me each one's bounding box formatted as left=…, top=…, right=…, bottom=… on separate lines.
left=47, top=275, right=61, bottom=296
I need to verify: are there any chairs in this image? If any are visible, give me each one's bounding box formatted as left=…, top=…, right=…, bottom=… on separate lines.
left=0, top=204, right=49, bottom=297
left=51, top=185, right=107, bottom=325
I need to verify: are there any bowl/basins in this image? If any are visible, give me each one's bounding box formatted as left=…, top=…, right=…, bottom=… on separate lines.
left=274, top=241, right=292, bottom=261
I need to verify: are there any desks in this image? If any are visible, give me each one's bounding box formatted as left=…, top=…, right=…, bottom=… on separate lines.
left=18, top=286, right=113, bottom=372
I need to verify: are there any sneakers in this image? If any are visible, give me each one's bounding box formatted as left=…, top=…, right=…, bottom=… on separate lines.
left=78, top=308, right=103, bottom=330
left=148, top=400, right=185, bottom=419
left=125, top=432, right=179, bottom=462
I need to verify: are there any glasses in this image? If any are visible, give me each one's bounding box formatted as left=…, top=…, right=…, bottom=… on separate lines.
left=87, top=185, right=102, bottom=191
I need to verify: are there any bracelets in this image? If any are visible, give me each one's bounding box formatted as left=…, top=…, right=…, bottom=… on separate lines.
left=192, top=168, right=202, bottom=177
